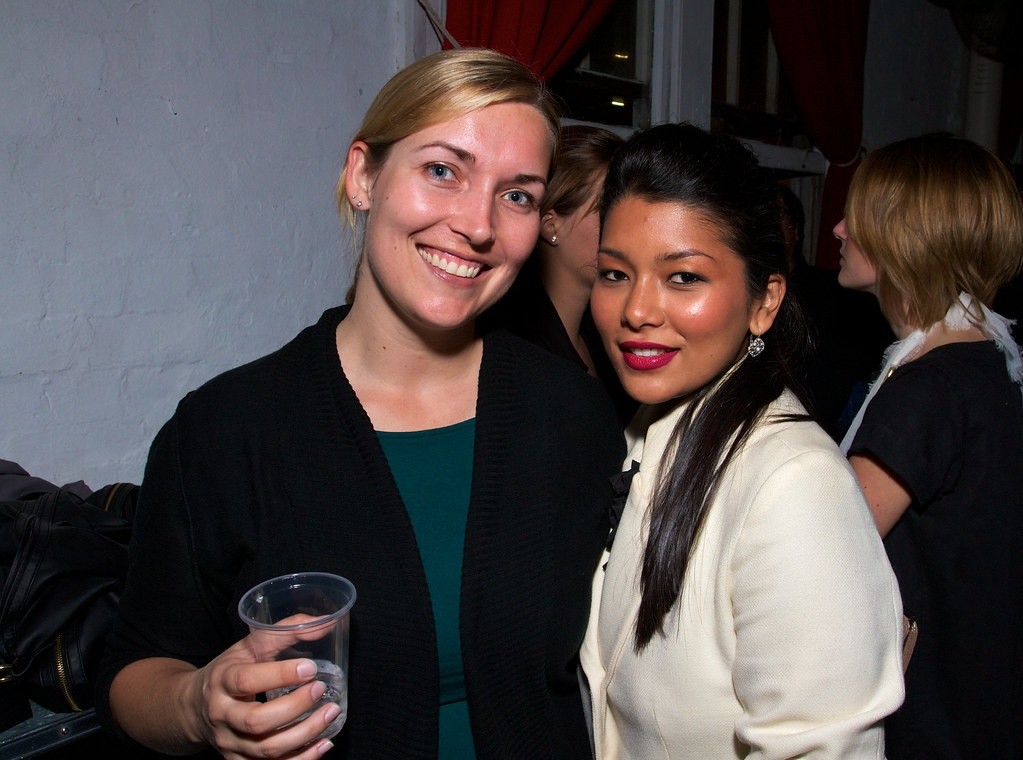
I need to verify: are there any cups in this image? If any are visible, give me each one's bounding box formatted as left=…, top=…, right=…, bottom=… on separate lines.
left=238, top=571, right=356, bottom=745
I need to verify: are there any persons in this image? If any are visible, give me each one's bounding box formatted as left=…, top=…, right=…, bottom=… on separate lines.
left=86, top=48, right=610, bottom=760
left=535, top=124, right=638, bottom=431
left=775, top=182, right=838, bottom=415
left=834, top=130, right=1023, bottom=760
left=578, top=121, right=906, bottom=759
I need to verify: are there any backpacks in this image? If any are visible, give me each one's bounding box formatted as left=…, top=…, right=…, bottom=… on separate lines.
left=0, top=461, right=140, bottom=734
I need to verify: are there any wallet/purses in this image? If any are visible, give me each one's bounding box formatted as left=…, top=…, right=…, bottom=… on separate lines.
left=902, top=613, right=919, bottom=675
